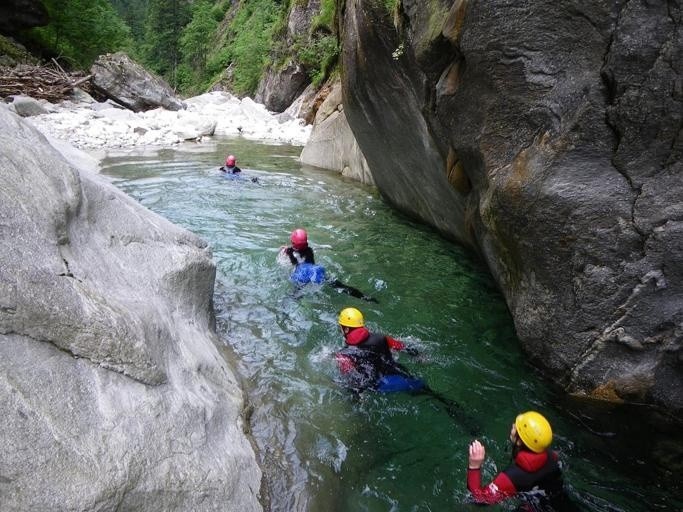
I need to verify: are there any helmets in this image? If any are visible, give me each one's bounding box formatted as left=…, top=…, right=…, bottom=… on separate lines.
left=227, top=155, right=236, bottom=167
left=291, top=229, right=308, bottom=245
left=338, top=308, right=365, bottom=328
left=516, top=411, right=553, bottom=454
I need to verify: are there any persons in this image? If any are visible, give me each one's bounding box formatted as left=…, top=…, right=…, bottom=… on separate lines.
left=218, top=154, right=257, bottom=185
left=327, top=306, right=493, bottom=468
left=273, top=228, right=380, bottom=317
left=462, top=409, right=595, bottom=510
